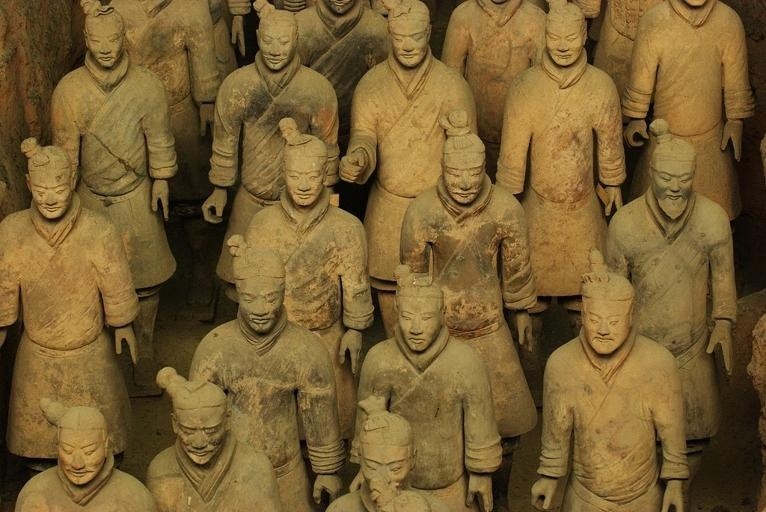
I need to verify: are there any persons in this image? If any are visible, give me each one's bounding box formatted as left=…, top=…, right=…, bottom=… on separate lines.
left=0, top=0, right=766, bottom=511
